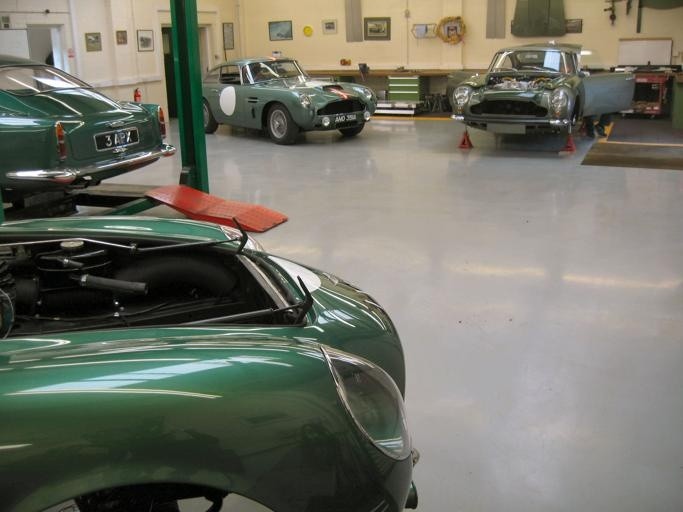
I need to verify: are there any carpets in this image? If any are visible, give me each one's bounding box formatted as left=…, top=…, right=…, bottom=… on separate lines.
left=581, top=117, right=683, bottom=170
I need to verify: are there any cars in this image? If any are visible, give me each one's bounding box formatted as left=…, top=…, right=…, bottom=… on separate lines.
left=2, top=53, right=177, bottom=188
left=201, top=56, right=378, bottom=145
left=450, top=38, right=636, bottom=138
left=1, top=215, right=420, bottom=512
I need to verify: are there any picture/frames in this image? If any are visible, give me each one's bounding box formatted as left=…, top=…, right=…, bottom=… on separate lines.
left=321, top=19, right=337, bottom=34
left=85, top=32, right=102, bottom=52
left=137, top=29, right=155, bottom=52
left=268, top=21, right=292, bottom=41
left=364, top=17, right=391, bottom=41
left=116, top=30, right=127, bottom=44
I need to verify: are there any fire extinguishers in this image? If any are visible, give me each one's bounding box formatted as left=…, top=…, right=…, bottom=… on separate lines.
left=134, top=87, right=142, bottom=102
